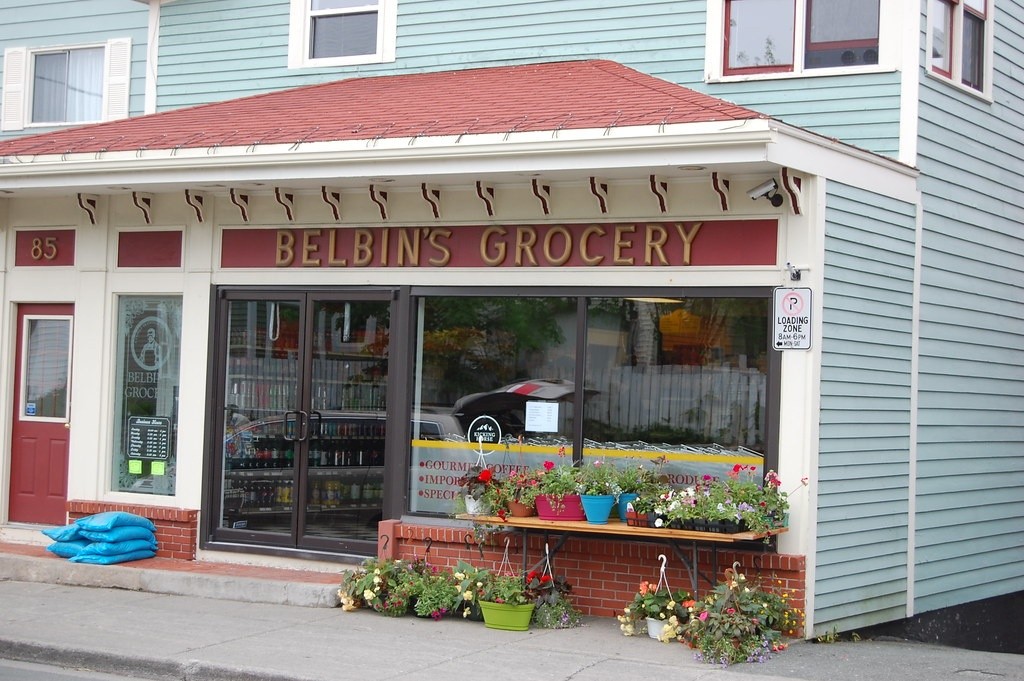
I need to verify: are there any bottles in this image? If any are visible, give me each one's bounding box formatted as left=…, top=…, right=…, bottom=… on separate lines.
left=227, top=441, right=380, bottom=468
left=224, top=474, right=383, bottom=511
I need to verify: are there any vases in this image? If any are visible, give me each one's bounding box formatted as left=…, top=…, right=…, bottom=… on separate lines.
left=669, top=518, right=682, bottom=529
left=462, top=600, right=485, bottom=622
left=579, top=494, right=615, bottom=524
left=477, top=599, right=536, bottom=631
left=647, top=513, right=671, bottom=528
left=505, top=500, right=536, bottom=517
left=372, top=590, right=389, bottom=612
left=617, top=493, right=640, bottom=523
left=645, top=617, right=669, bottom=638
left=535, top=494, right=559, bottom=520
left=625, top=512, right=638, bottom=526
left=761, top=513, right=789, bottom=530
left=682, top=519, right=694, bottom=530
left=411, top=597, right=433, bottom=618
left=638, top=515, right=648, bottom=527
left=724, top=519, right=751, bottom=534
left=464, top=495, right=492, bottom=515
left=707, top=520, right=725, bottom=533
left=556, top=494, right=586, bottom=520
left=732, top=638, right=740, bottom=649
left=694, top=519, right=709, bottom=531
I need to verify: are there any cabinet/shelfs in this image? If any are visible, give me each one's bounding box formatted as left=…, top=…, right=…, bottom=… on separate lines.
left=227, top=343, right=388, bottom=526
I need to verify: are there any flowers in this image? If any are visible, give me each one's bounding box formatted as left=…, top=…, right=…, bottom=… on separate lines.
left=339, top=446, right=806, bottom=670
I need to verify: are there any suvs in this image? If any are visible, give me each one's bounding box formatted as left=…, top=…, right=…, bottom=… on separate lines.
left=133, top=375, right=602, bottom=525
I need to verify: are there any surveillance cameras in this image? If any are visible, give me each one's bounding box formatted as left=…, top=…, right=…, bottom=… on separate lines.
left=746, top=178, right=778, bottom=200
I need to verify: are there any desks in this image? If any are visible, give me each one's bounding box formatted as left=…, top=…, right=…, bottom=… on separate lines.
left=456, top=513, right=790, bottom=601
